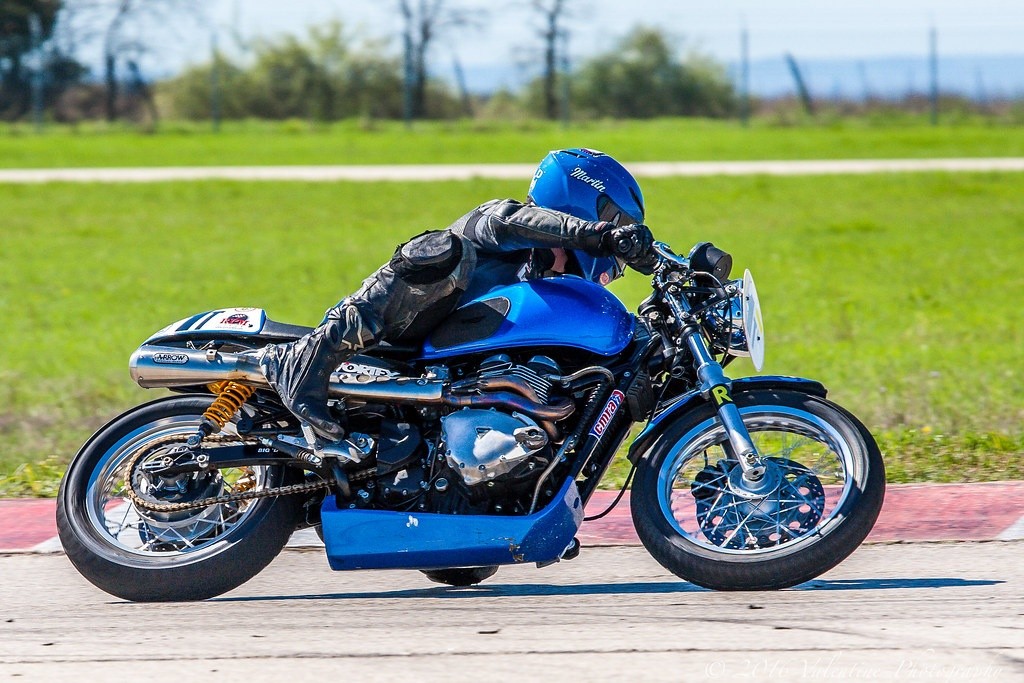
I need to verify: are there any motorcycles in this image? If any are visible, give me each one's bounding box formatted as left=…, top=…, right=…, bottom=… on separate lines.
left=56, top=235, right=887, bottom=604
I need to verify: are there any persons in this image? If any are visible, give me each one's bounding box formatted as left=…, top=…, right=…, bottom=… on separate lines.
left=259, top=148, right=664, bottom=588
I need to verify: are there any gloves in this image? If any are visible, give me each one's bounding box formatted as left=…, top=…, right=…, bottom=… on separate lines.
left=608, top=222, right=659, bottom=276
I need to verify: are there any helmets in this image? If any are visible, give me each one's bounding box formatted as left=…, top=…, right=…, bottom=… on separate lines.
left=528, top=147, right=646, bottom=285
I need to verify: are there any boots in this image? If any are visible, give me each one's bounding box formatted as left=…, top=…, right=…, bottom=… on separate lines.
left=259, top=291, right=385, bottom=442
